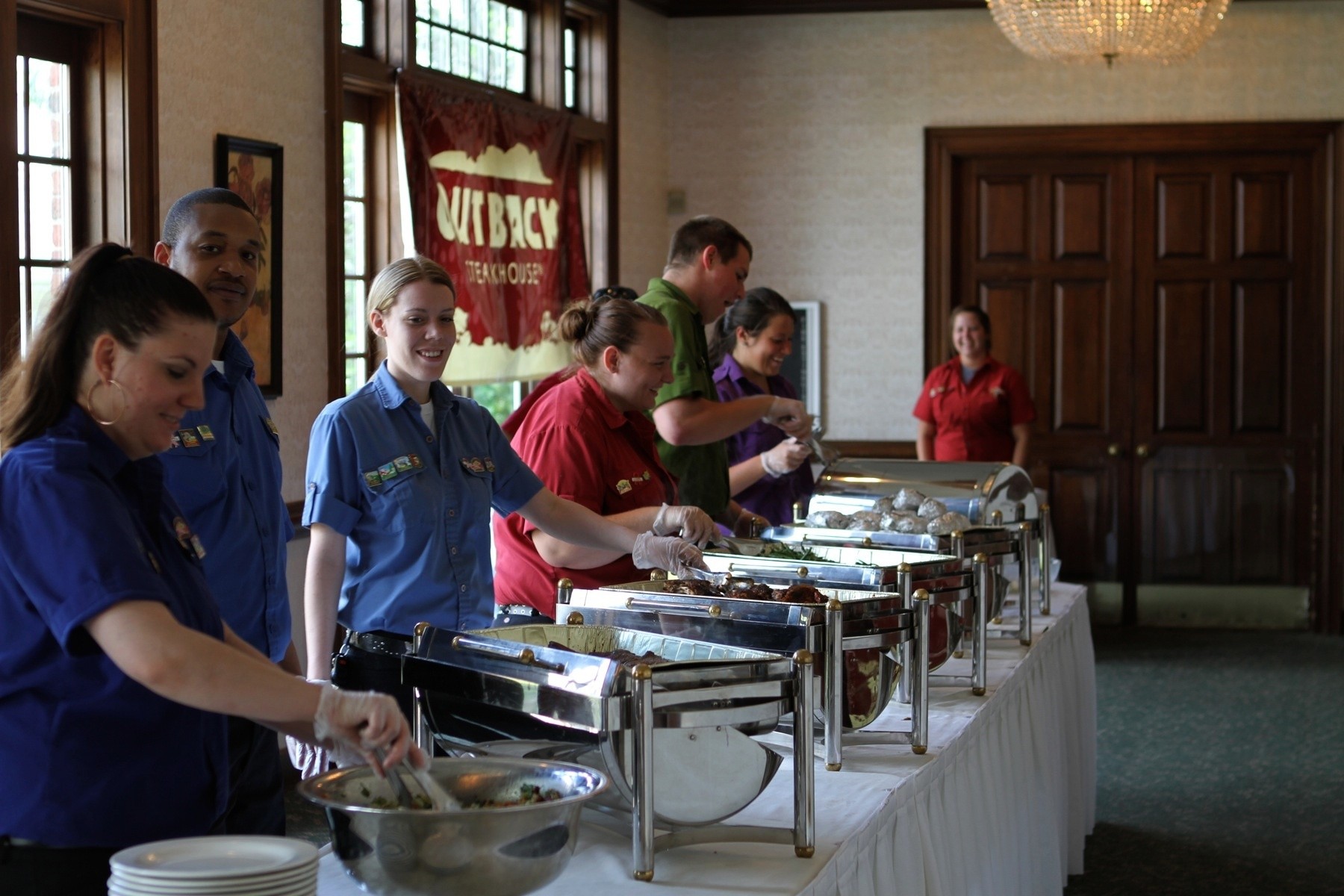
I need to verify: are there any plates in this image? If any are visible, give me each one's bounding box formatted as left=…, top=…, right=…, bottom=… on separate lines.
left=459, top=739, right=611, bottom=776
left=105, top=835, right=319, bottom=896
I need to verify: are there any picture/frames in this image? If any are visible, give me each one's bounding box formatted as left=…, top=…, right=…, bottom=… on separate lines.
left=213, top=132, right=286, bottom=398
left=721, top=300, right=822, bottom=434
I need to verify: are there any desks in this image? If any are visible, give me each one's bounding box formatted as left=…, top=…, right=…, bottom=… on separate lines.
left=317, top=582, right=1099, bottom=896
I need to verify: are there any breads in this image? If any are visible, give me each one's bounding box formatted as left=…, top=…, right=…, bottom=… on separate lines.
left=804, top=487, right=972, bottom=535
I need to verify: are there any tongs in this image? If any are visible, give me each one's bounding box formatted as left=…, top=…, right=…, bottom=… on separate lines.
left=678, top=527, right=766, bottom=556
left=682, top=564, right=754, bottom=596
left=356, top=722, right=464, bottom=812
left=779, top=416, right=840, bottom=468
left=520, top=741, right=599, bottom=765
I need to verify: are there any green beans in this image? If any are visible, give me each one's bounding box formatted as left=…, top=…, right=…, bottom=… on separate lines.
left=756, top=542, right=879, bottom=567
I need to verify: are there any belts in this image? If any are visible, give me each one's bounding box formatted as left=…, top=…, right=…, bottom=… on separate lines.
left=337, top=625, right=412, bottom=657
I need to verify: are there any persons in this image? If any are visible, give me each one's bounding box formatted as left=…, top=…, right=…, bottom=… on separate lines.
left=0, top=236, right=433, bottom=896
left=106, top=188, right=302, bottom=834
left=477, top=287, right=754, bottom=636
left=500, top=288, right=636, bottom=438
left=702, top=287, right=828, bottom=543
left=627, top=214, right=840, bottom=544
left=288, top=259, right=711, bottom=792
left=909, top=305, right=1034, bottom=472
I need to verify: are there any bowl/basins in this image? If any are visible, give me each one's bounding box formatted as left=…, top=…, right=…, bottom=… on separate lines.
left=297, top=756, right=610, bottom=896
left=1000, top=557, right=1061, bottom=596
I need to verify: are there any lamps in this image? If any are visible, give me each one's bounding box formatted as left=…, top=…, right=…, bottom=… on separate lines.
left=984, top=0, right=1234, bottom=70
